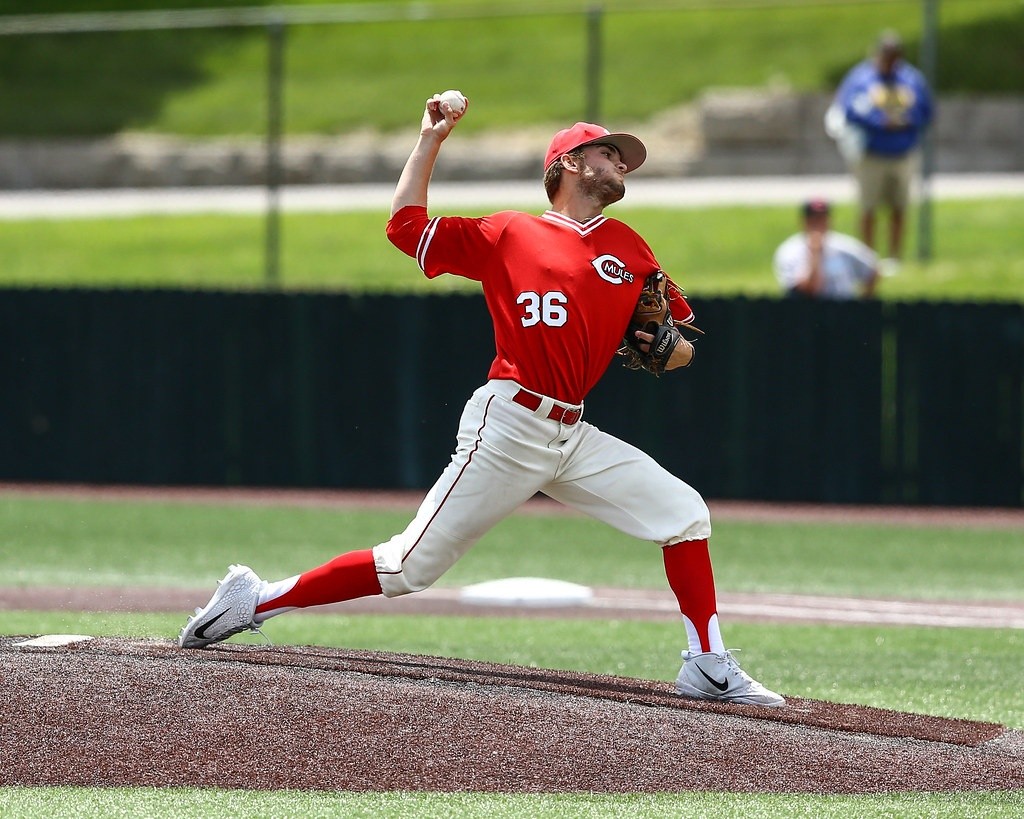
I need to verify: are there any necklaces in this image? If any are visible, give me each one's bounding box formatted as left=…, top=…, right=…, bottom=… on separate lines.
left=581, top=216, right=593, bottom=223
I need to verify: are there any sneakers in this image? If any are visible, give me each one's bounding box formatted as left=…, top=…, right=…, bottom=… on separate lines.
left=179, top=564, right=272, bottom=650
left=675, top=649, right=786, bottom=708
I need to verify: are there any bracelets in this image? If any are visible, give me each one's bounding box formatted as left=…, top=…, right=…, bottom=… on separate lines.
left=683, top=343, right=695, bottom=368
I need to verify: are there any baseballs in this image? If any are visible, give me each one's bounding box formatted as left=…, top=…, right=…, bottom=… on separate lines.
left=437, top=89, right=466, bottom=118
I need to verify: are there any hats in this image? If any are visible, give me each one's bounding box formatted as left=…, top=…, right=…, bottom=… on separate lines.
left=545, top=123, right=648, bottom=176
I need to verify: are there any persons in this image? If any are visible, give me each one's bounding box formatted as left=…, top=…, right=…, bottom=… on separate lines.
left=823, top=28, right=934, bottom=260
left=173, top=88, right=790, bottom=711
left=770, top=199, right=900, bottom=303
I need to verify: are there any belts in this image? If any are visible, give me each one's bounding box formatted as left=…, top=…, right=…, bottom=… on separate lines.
left=513, top=389, right=581, bottom=424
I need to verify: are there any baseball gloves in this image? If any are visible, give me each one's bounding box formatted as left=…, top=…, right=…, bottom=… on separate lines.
left=615, top=269, right=707, bottom=380
